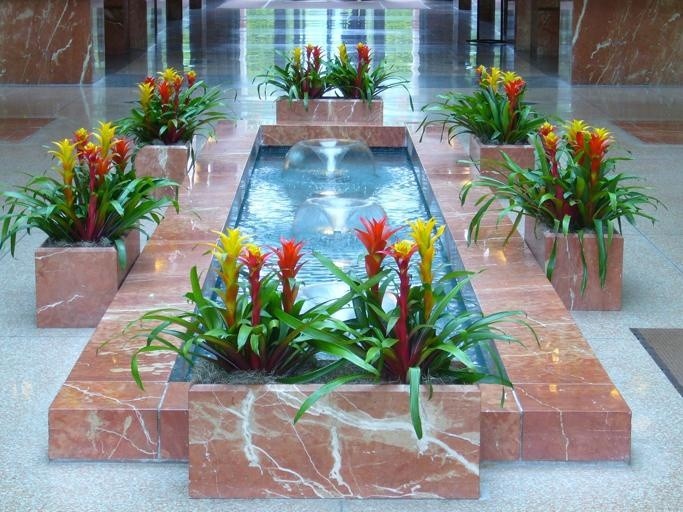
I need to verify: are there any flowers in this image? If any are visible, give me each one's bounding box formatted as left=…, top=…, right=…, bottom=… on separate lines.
left=111, top=66, right=241, bottom=183
left=288, top=214, right=540, bottom=441
left=1, top=119, right=203, bottom=271
left=251, top=40, right=415, bottom=112
left=413, top=64, right=568, bottom=147
left=459, top=118, right=669, bottom=298
left=95, top=219, right=364, bottom=403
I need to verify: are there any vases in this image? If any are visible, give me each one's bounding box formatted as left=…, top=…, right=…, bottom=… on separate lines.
left=36, top=218, right=141, bottom=328
left=132, top=136, right=198, bottom=201
left=187, top=358, right=481, bottom=501
left=277, top=95, right=384, bottom=126
left=468, top=134, right=537, bottom=195
left=525, top=210, right=624, bottom=311
